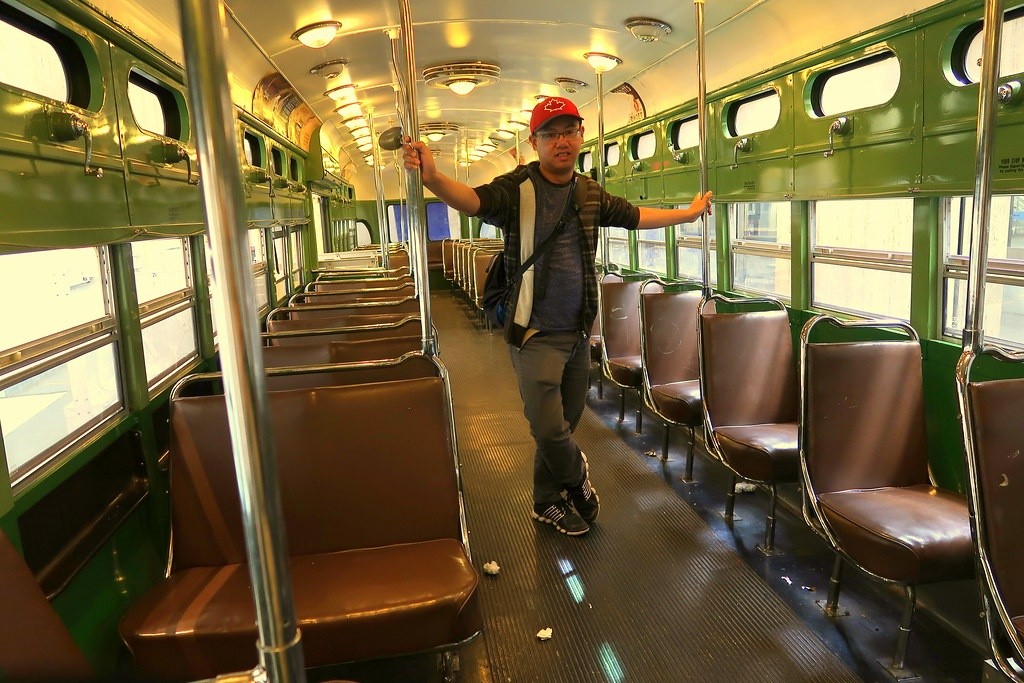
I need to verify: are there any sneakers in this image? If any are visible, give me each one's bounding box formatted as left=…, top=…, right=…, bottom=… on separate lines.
left=572, top=450, right=601, bottom=523
left=530, top=497, right=590, bottom=536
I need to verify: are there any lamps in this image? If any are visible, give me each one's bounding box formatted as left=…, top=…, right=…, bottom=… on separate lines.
left=625, top=20, right=670, bottom=44
left=309, top=59, right=349, bottom=81
left=557, top=78, right=588, bottom=93
left=583, top=53, right=623, bottom=73
left=321, top=80, right=549, bottom=171
left=290, top=21, right=343, bottom=50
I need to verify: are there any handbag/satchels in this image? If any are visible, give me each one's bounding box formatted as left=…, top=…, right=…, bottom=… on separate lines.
left=481, top=250, right=516, bottom=330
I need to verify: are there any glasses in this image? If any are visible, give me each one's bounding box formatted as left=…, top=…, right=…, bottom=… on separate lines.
left=535, top=129, right=582, bottom=142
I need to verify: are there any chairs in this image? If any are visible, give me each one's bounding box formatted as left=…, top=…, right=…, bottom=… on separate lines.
left=441, top=236, right=1024, bottom=682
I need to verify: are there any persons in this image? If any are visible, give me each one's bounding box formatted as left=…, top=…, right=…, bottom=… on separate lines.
left=403, top=95, right=714, bottom=538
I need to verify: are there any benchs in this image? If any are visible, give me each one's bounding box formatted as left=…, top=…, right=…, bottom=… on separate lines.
left=1, top=236, right=484, bottom=683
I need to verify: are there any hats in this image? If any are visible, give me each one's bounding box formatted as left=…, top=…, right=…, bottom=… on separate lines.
left=530, top=96, right=584, bottom=135
